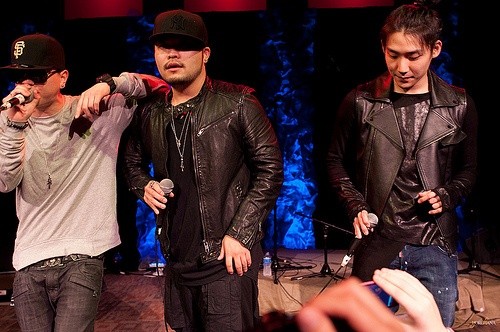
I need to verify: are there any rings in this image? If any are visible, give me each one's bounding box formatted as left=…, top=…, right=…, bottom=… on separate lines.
left=9, top=91, right=15, bottom=97
left=149, top=181, right=155, bottom=187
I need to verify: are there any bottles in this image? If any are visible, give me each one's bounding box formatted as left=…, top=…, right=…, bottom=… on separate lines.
left=115, top=252, right=122, bottom=266
left=263, top=252, right=272, bottom=277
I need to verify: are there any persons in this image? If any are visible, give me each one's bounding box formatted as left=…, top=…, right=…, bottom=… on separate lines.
left=0, top=34, right=172, bottom=332
left=263, top=268, right=455, bottom=332
left=324, top=4, right=477, bottom=330
left=116, top=9, right=283, bottom=332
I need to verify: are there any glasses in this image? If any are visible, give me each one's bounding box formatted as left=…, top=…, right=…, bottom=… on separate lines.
left=7, top=69, right=63, bottom=84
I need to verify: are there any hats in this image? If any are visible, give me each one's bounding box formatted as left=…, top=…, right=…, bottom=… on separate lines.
left=149, top=9, right=208, bottom=47
left=0, top=33, right=65, bottom=69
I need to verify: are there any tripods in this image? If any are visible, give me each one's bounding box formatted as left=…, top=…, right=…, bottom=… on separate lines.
left=259, top=203, right=313, bottom=284
left=294, top=210, right=353, bottom=281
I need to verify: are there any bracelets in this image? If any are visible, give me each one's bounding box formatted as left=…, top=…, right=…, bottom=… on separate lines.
left=0, top=111, right=29, bottom=130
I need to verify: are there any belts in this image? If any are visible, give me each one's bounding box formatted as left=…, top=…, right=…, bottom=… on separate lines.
left=32, top=254, right=103, bottom=268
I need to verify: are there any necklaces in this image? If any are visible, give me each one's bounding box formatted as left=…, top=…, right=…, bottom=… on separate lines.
left=31, top=95, right=64, bottom=189
left=168, top=95, right=198, bottom=171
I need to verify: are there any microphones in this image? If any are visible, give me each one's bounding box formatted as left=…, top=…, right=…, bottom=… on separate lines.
left=0, top=90, right=34, bottom=110
left=156, top=178, right=174, bottom=235
left=342, top=212, right=379, bottom=267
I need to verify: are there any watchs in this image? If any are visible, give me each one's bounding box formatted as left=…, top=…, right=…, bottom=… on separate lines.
left=95, top=73, right=116, bottom=94
